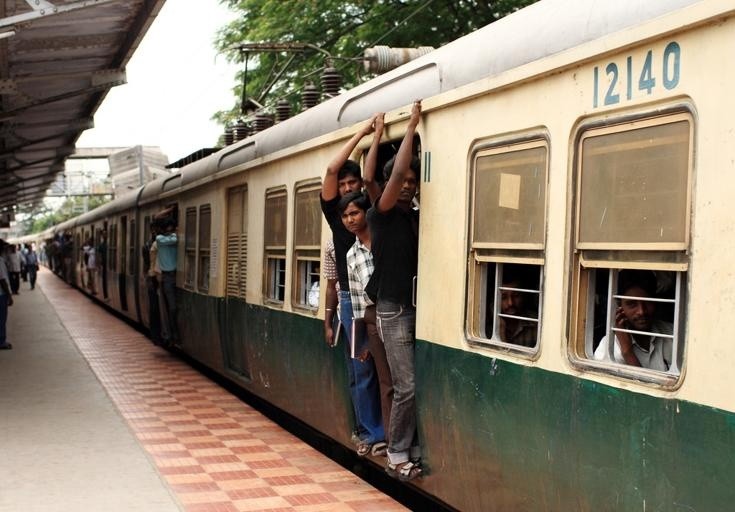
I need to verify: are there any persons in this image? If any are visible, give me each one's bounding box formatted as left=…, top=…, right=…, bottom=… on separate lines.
left=499, top=284, right=537, bottom=348
left=142, top=206, right=179, bottom=345
left=0, top=237, right=42, bottom=350
left=42, top=230, right=108, bottom=299
left=309, top=267, right=320, bottom=308
left=594, top=269, right=676, bottom=373
left=319, top=97, right=422, bottom=483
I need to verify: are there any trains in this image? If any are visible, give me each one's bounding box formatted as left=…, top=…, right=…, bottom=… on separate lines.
left=10, top=0, right=735, bottom=512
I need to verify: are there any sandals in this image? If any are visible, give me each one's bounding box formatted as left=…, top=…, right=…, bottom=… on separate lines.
left=357, top=443, right=421, bottom=481
